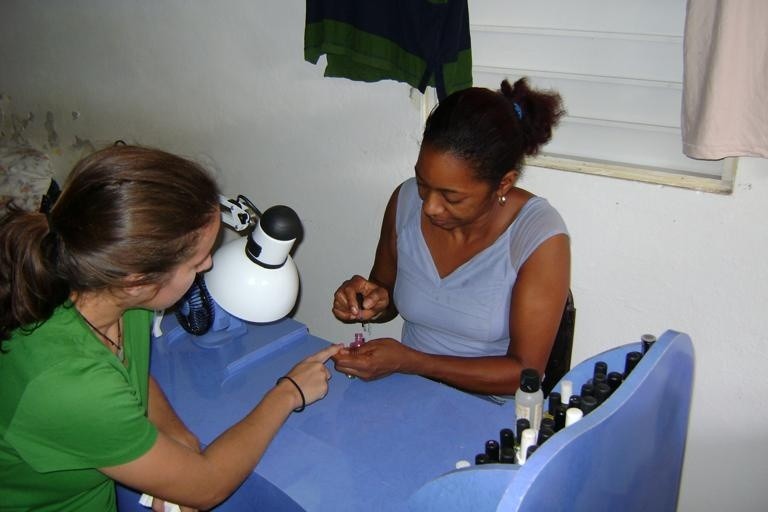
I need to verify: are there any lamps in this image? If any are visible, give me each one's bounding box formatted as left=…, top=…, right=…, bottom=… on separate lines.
left=203, top=188, right=304, bottom=326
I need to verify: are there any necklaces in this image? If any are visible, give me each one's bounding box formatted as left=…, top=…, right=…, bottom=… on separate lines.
left=74, top=310, right=127, bottom=364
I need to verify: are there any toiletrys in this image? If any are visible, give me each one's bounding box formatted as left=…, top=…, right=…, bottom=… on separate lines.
left=454, top=332, right=657, bottom=471
left=348, top=331, right=367, bottom=350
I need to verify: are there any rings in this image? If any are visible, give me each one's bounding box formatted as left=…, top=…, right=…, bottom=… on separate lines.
left=347, top=372, right=354, bottom=378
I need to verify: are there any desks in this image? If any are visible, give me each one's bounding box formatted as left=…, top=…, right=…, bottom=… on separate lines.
left=116, top=297, right=696, bottom=512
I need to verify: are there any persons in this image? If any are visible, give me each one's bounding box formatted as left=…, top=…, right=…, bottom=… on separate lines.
left=0, top=139, right=346, bottom=512
left=325, top=74, right=574, bottom=398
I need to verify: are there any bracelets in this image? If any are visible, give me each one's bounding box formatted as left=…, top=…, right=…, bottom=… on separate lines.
left=276, top=375, right=306, bottom=414
